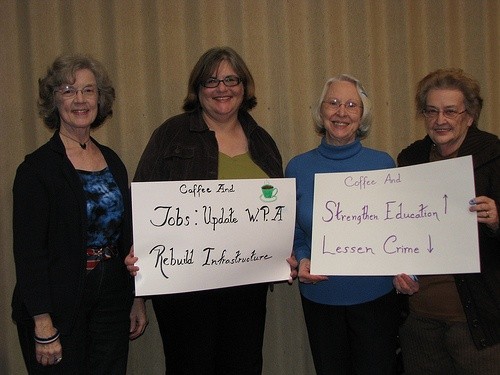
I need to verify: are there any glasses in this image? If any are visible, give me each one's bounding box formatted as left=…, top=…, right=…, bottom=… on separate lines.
left=54, top=86, right=99, bottom=97
left=323, top=98, right=362, bottom=110
left=200, top=75, right=242, bottom=88
left=422, top=105, right=467, bottom=119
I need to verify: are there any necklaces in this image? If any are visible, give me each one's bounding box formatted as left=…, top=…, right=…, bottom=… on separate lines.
left=59, top=132, right=90, bottom=150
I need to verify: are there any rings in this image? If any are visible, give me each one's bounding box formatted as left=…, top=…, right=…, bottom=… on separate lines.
left=486, top=211, right=489, bottom=218
left=54, top=357, right=63, bottom=363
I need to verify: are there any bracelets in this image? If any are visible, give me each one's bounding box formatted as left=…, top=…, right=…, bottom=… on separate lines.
left=34, top=329, right=60, bottom=344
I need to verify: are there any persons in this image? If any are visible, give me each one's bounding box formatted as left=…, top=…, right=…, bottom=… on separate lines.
left=287, top=74, right=420, bottom=374
left=11, top=54, right=149, bottom=375
left=397, top=68, right=500, bottom=375
left=124, top=47, right=299, bottom=375
